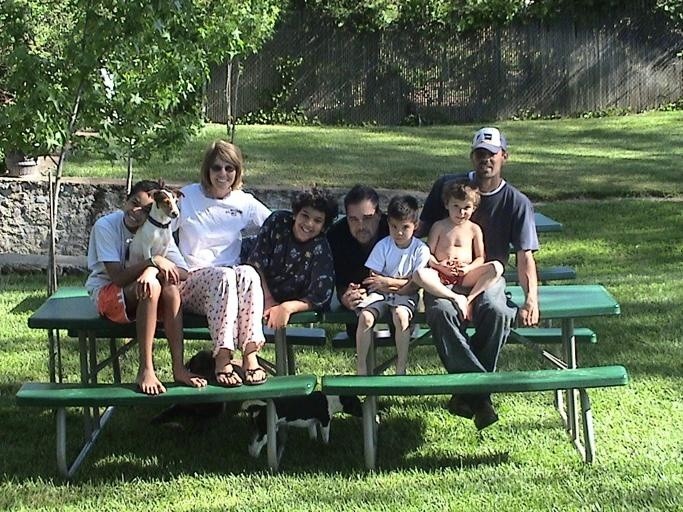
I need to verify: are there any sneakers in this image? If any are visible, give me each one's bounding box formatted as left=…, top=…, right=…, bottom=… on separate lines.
left=449, top=396, right=497, bottom=430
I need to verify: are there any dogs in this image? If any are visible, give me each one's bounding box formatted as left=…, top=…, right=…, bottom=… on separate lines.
left=148, top=349, right=227, bottom=433
left=123, top=188, right=186, bottom=269
left=240, top=390, right=364, bottom=459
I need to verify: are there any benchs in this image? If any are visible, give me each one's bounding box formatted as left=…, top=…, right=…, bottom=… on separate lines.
left=14, top=209, right=626, bottom=475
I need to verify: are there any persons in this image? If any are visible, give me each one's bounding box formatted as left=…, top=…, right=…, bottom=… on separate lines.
left=172, top=140, right=272, bottom=388
left=85, top=181, right=207, bottom=396
left=239, top=183, right=339, bottom=330
left=413, top=127, right=540, bottom=427
left=326, top=185, right=429, bottom=376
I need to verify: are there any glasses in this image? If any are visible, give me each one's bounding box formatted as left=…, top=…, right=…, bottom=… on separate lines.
left=212, top=165, right=234, bottom=172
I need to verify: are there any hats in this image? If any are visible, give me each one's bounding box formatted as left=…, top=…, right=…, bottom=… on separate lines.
left=472, top=126, right=506, bottom=154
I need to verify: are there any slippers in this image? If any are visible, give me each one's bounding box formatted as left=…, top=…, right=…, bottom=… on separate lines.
left=244, top=367, right=267, bottom=385
left=215, top=371, right=243, bottom=387
